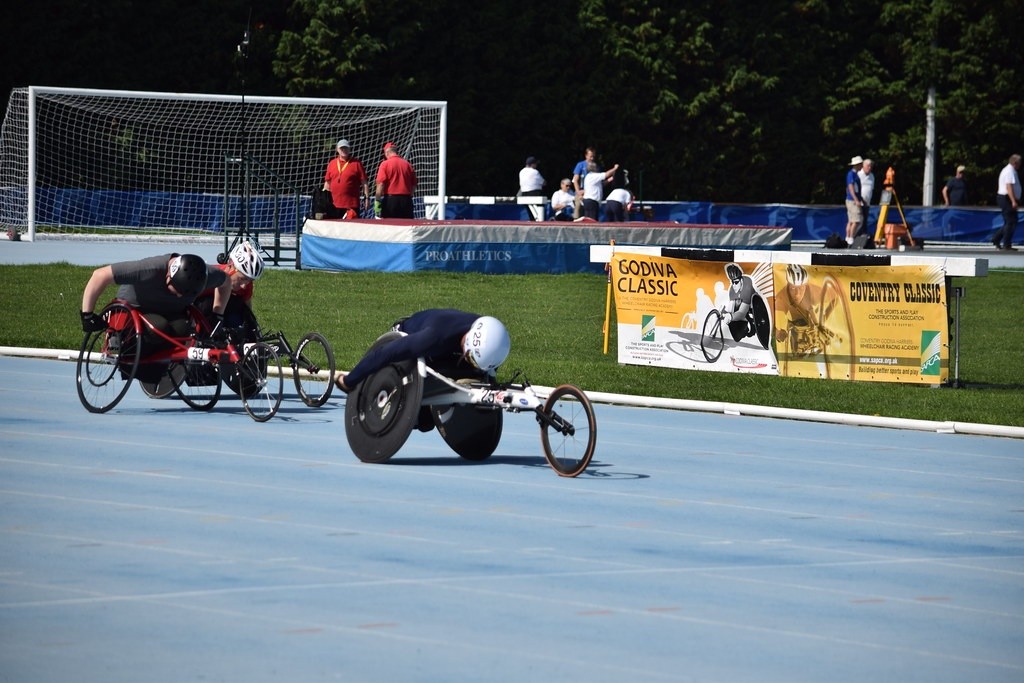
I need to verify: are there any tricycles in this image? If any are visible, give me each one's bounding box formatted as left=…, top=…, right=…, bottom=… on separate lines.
left=345, top=328, right=596, bottom=477
left=75, top=297, right=335, bottom=423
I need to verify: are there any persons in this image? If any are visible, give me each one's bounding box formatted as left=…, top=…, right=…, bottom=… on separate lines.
left=942, top=165, right=973, bottom=207
left=516, top=148, right=639, bottom=222
left=991, top=154, right=1022, bottom=252
left=376, top=142, right=417, bottom=220
left=332, top=308, right=510, bottom=402
left=323, top=139, right=370, bottom=219
left=82, top=241, right=264, bottom=368
left=844, top=156, right=876, bottom=243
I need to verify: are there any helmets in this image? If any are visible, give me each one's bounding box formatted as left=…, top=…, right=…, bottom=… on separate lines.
left=229, top=241, right=265, bottom=280
left=170, top=254, right=206, bottom=297
left=465, top=316, right=510, bottom=372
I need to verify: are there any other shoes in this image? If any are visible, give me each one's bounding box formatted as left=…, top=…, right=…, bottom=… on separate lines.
left=1004, top=246, right=1019, bottom=251
left=991, top=240, right=1001, bottom=249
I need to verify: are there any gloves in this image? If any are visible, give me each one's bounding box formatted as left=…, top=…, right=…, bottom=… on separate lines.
left=79, top=309, right=109, bottom=332
left=208, top=312, right=224, bottom=335
left=334, top=374, right=350, bottom=395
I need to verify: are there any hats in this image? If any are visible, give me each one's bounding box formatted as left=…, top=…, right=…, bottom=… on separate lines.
left=526, top=156, right=539, bottom=163
left=384, top=142, right=397, bottom=153
left=848, top=156, right=865, bottom=165
left=337, top=138, right=350, bottom=148
